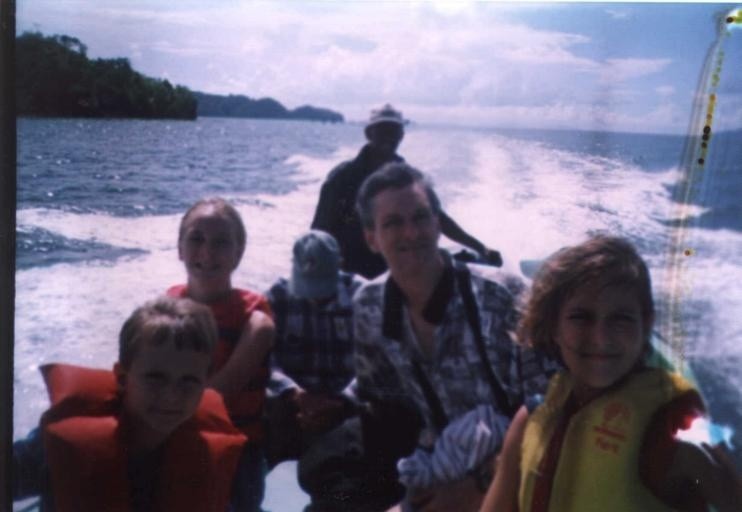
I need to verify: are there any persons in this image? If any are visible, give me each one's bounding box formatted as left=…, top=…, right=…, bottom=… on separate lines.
left=12, top=101, right=741, bottom=511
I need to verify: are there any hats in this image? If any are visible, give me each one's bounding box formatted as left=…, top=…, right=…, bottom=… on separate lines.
left=365, top=108, right=403, bottom=130
